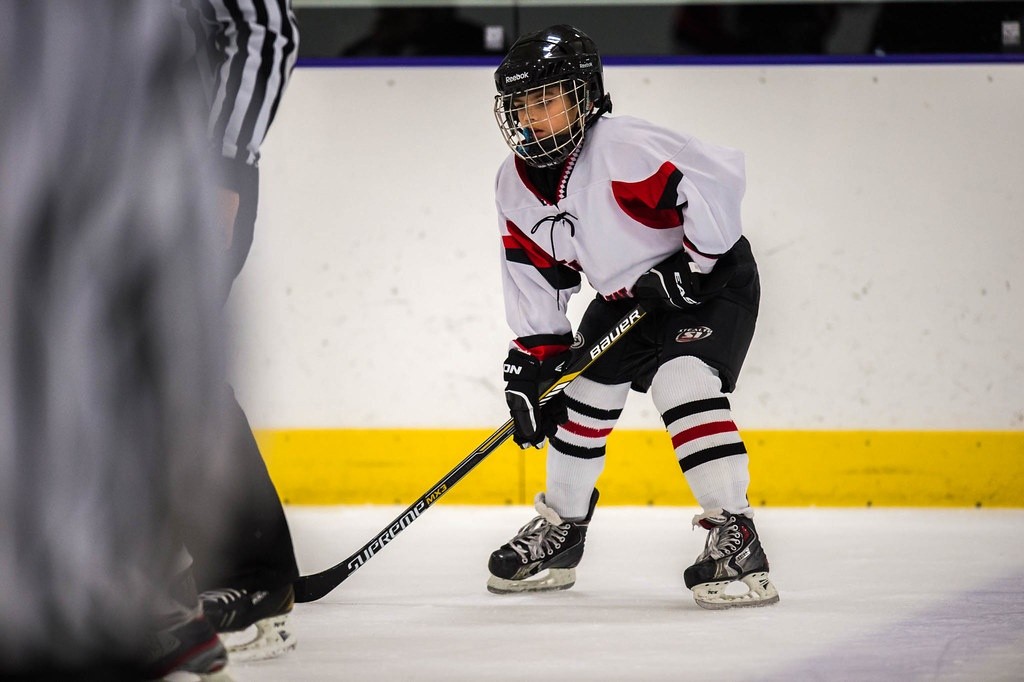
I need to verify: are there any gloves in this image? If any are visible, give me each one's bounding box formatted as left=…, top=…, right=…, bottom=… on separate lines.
left=504, top=348, right=569, bottom=448
left=630, top=248, right=712, bottom=321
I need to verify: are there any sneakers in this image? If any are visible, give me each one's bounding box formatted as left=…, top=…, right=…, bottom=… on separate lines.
left=198, top=567, right=297, bottom=663
left=684, top=506, right=780, bottom=609
left=487, top=488, right=600, bottom=592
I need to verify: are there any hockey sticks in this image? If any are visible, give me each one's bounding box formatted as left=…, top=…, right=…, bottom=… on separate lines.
left=291, top=303, right=648, bottom=604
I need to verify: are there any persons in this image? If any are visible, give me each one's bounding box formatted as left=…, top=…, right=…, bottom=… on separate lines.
left=0, top=0, right=303, bottom=682
left=669, top=3, right=1024, bottom=56
left=339, top=8, right=507, bottom=58
left=486, top=22, right=780, bottom=611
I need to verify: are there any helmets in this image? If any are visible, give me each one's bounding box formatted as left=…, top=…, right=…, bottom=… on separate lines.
left=495, top=23, right=612, bottom=168
